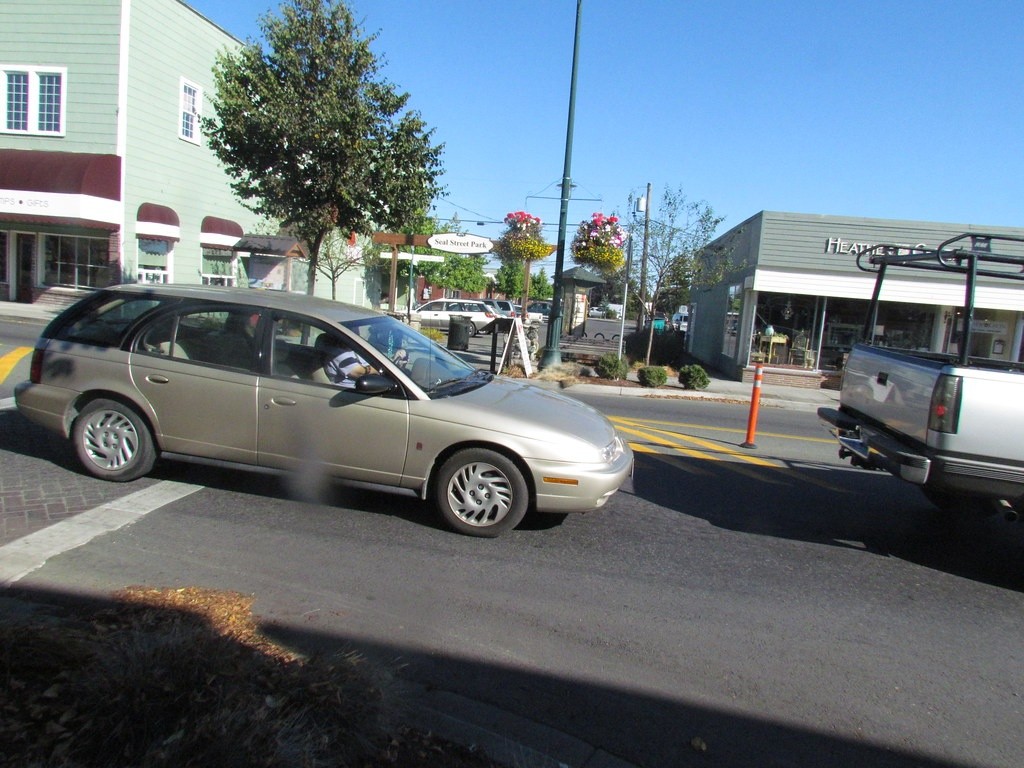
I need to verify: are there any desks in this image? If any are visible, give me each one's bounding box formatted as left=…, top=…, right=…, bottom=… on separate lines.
left=758, top=336, right=786, bottom=364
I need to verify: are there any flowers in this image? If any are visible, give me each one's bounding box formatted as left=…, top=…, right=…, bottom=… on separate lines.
left=490, top=212, right=551, bottom=259
left=571, top=213, right=628, bottom=272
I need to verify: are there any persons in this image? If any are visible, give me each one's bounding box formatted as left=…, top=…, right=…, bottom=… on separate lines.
left=323, top=327, right=406, bottom=389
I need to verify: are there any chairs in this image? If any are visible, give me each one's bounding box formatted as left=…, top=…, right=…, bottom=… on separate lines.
left=750, top=338, right=766, bottom=364
left=308, top=331, right=335, bottom=385
left=787, top=338, right=809, bottom=367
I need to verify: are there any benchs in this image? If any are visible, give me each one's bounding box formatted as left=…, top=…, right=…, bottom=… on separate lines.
left=159, top=328, right=258, bottom=373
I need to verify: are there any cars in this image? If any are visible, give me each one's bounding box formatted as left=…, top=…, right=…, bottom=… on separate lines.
left=400, top=298, right=563, bottom=345
left=671, top=312, right=689, bottom=332
left=589, top=306, right=611, bottom=319
left=14, top=282, right=634, bottom=538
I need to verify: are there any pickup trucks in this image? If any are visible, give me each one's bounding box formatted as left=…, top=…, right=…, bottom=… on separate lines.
left=817, top=231, right=1024, bottom=524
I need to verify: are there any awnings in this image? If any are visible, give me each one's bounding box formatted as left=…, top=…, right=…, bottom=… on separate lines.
left=135, top=202, right=180, bottom=242
left=200, top=216, right=243, bottom=251
left=0, top=148, right=122, bottom=231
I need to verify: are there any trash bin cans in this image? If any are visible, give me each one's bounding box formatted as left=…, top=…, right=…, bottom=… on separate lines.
left=654, top=319, right=665, bottom=334
left=447, top=314, right=471, bottom=350
